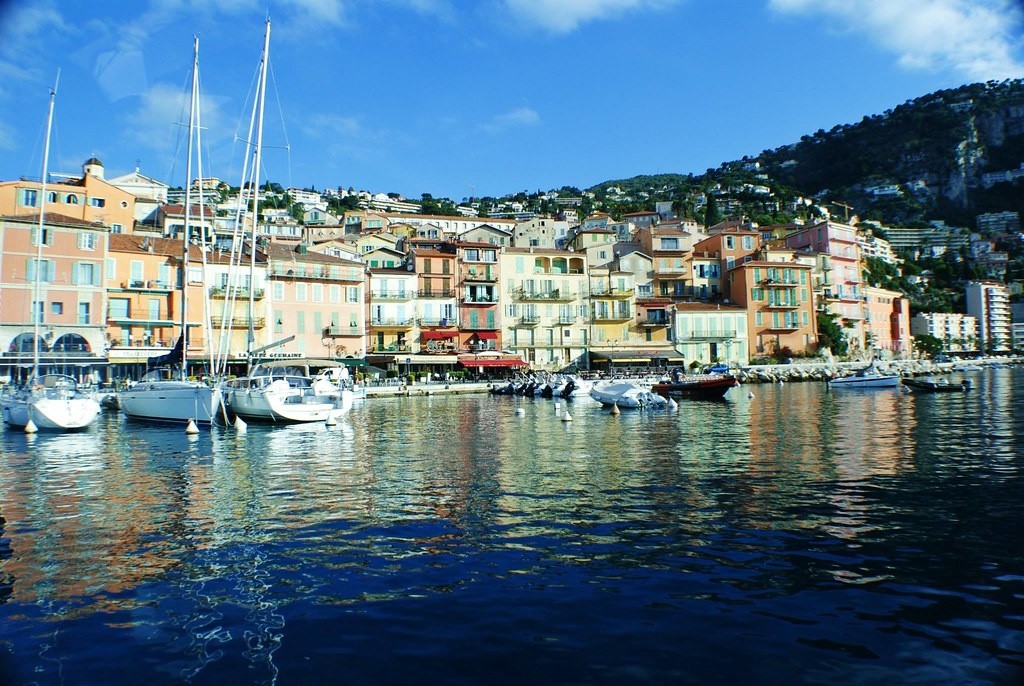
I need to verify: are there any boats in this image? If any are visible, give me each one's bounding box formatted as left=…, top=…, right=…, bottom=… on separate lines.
left=740, top=367, right=850, bottom=383
left=901, top=378, right=976, bottom=393
left=490, top=370, right=581, bottom=396
left=952, top=362, right=1010, bottom=372
left=589, top=377, right=668, bottom=408
left=877, top=361, right=952, bottom=377
left=651, top=374, right=737, bottom=400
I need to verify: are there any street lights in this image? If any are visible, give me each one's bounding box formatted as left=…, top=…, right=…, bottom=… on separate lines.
left=321, top=336, right=336, bottom=378
left=607, top=339, right=619, bottom=379
left=469, top=338, right=482, bottom=383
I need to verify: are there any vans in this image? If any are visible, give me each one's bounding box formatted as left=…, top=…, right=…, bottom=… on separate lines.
left=317, top=368, right=349, bottom=380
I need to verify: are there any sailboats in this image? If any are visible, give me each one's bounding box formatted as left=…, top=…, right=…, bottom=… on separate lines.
left=122, top=38, right=221, bottom=426
left=0, top=67, right=100, bottom=428
left=830, top=293, right=900, bottom=388
left=220, top=16, right=353, bottom=421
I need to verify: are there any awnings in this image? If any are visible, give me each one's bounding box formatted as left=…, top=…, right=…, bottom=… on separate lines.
left=422, top=331, right=459, bottom=339
left=457, top=360, right=528, bottom=367
left=475, top=332, right=498, bottom=339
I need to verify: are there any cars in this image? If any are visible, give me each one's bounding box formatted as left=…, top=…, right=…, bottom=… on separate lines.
left=935, top=355, right=951, bottom=363
left=415, top=372, right=433, bottom=381
left=706, top=364, right=729, bottom=374
left=482, top=373, right=501, bottom=379
